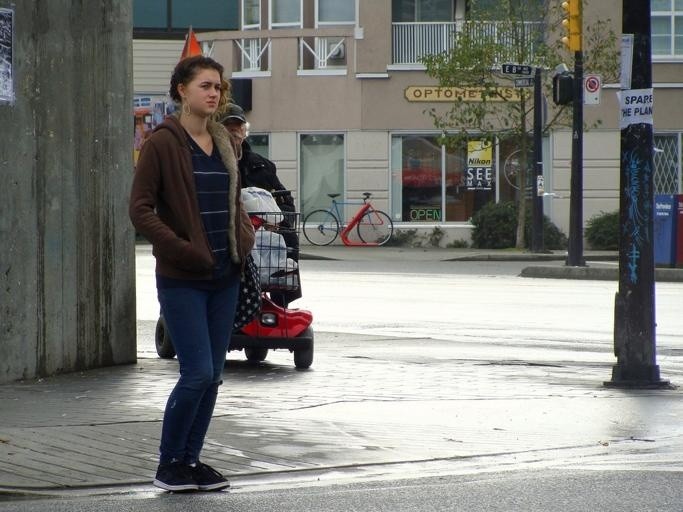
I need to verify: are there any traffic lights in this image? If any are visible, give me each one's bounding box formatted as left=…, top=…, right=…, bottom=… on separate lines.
left=560, top=0, right=582, bottom=53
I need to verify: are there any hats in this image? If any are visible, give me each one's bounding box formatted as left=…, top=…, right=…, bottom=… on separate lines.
left=214, top=103, right=246, bottom=123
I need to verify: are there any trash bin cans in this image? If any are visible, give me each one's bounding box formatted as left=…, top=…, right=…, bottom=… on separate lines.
left=653, top=194, right=683, bottom=268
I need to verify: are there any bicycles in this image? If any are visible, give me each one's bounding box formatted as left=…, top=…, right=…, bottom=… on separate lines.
left=301, top=192, right=393, bottom=247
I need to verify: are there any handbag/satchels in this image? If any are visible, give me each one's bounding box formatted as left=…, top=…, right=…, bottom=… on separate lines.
left=231, top=252, right=262, bottom=331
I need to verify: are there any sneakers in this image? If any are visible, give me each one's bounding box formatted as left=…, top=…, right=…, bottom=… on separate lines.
left=153, top=460, right=230, bottom=492
